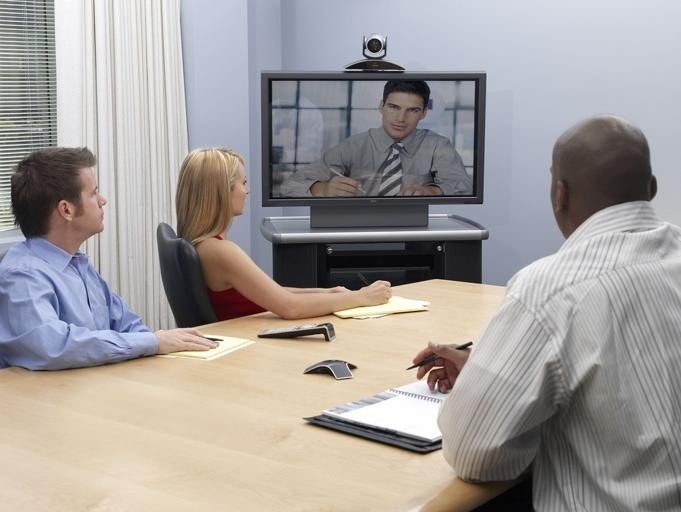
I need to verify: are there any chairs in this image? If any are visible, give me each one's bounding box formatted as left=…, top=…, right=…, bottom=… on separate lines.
left=157, top=222, right=220, bottom=328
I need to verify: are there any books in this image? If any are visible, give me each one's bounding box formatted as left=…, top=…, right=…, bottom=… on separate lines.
left=161, top=331, right=254, bottom=358
left=302, top=380, right=455, bottom=454
left=331, top=296, right=431, bottom=320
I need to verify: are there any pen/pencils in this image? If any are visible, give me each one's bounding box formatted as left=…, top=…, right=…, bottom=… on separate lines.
left=357, top=274, right=371, bottom=286
left=206, top=338, right=224, bottom=341
left=329, top=168, right=366, bottom=193
left=406, top=342, right=473, bottom=370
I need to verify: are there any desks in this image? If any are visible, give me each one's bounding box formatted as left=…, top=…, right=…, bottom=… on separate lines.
left=0, top=279, right=532, bottom=512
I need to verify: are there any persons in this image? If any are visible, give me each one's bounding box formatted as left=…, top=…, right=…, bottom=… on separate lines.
left=279, top=78, right=472, bottom=198
left=0, top=148, right=221, bottom=371
left=413, top=116, right=680, bottom=512
left=176, top=147, right=390, bottom=320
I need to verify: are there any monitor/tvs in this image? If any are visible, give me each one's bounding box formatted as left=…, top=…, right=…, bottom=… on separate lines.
left=258, top=69, right=488, bottom=228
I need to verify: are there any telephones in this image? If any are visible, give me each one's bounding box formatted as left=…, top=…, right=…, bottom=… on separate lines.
left=303, top=359, right=357, bottom=379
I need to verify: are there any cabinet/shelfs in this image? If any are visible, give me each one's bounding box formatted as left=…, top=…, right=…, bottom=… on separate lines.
left=260, top=213, right=489, bottom=290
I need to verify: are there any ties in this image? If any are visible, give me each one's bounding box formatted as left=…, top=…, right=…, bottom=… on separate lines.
left=378, top=142, right=403, bottom=196
